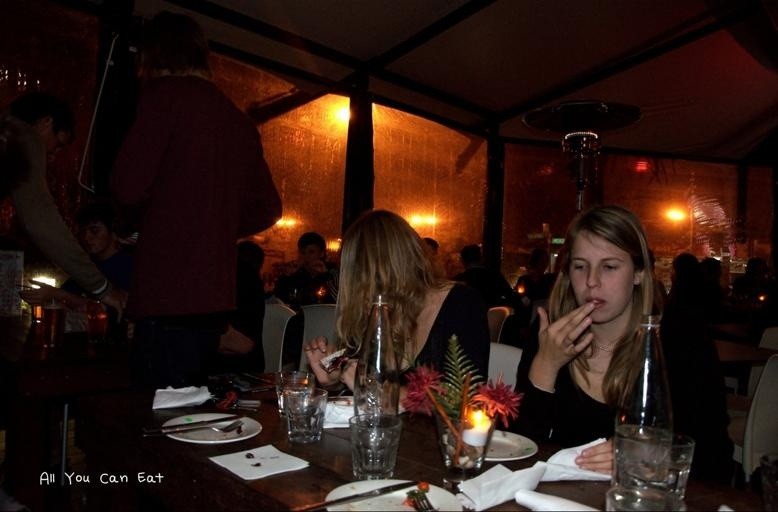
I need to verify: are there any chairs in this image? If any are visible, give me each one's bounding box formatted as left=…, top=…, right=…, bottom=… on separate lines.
left=742, top=354, right=778, bottom=476
left=747, top=327, right=778, bottom=396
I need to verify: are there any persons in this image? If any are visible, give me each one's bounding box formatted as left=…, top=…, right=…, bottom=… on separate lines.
left=303, top=211, right=492, bottom=392
left=233, top=224, right=778, bottom=400
left=109, top=11, right=282, bottom=379
left=0, top=94, right=128, bottom=325
left=512, top=206, right=736, bottom=485
left=0, top=181, right=132, bottom=321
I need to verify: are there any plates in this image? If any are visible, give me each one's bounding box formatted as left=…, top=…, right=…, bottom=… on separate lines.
left=320, top=477, right=463, bottom=512
left=159, top=412, right=264, bottom=445
left=323, top=396, right=360, bottom=409
left=481, top=428, right=539, bottom=462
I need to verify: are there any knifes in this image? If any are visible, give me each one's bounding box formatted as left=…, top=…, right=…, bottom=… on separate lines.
left=289, top=480, right=422, bottom=512
left=143, top=411, right=247, bottom=434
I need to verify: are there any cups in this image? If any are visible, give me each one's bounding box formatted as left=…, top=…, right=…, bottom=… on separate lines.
left=606, top=420, right=695, bottom=510
left=273, top=367, right=318, bottom=421
left=282, top=386, right=327, bottom=444
left=348, top=413, right=401, bottom=479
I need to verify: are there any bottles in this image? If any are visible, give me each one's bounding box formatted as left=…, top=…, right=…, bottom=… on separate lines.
left=351, top=294, right=402, bottom=467
left=611, top=310, right=677, bottom=485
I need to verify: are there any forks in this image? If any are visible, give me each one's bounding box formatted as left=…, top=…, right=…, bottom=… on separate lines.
left=137, top=414, right=245, bottom=438
left=414, top=491, right=442, bottom=512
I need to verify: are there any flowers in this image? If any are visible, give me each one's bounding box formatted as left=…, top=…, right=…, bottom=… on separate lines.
left=401, top=333, right=527, bottom=462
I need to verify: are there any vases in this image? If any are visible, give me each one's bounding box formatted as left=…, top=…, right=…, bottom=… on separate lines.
left=436, top=416, right=493, bottom=481
